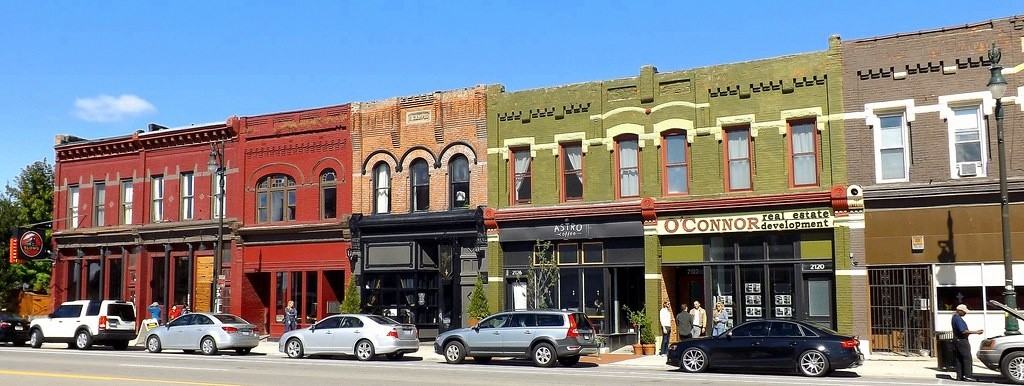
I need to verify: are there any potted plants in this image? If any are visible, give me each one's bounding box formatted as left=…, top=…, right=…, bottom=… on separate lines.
left=467, top=267, right=490, bottom=328
left=598, top=338, right=606, bottom=347
left=622, top=304, right=657, bottom=355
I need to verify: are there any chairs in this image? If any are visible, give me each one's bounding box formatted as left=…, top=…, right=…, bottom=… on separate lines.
left=197, top=317, right=204, bottom=324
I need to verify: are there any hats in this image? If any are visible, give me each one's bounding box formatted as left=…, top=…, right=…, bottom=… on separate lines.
left=957, top=304, right=969, bottom=313
left=152, top=302, right=158, bottom=306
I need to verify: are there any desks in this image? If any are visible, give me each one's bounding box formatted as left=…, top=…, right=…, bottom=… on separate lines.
left=587, top=316, right=605, bottom=334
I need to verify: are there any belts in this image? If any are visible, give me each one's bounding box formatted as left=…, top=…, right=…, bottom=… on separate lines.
left=694, top=325, right=701, bottom=328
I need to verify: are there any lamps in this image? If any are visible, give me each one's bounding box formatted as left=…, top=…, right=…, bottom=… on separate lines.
left=474, top=242, right=485, bottom=258
left=347, top=245, right=358, bottom=262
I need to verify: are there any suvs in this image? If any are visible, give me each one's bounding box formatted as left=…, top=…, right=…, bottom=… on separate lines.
left=28, top=299, right=138, bottom=349
left=433, top=307, right=598, bottom=367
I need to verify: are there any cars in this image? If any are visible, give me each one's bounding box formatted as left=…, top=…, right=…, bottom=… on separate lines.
left=279, top=312, right=421, bottom=362
left=0, top=310, right=31, bottom=344
left=975, top=299, right=1024, bottom=386
left=144, top=312, right=259, bottom=356
left=665, top=317, right=865, bottom=378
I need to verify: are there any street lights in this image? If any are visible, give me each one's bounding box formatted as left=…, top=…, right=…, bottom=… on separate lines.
left=989, top=40, right=1023, bottom=332
left=208, top=135, right=229, bottom=311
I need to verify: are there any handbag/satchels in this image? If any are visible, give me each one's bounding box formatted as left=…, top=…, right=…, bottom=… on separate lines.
left=283, top=318, right=285, bottom=323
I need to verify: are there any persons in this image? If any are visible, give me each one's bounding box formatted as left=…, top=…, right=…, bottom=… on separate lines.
left=712, top=301, right=729, bottom=337
left=172, top=294, right=191, bottom=315
left=285, top=300, right=297, bottom=333
left=689, top=300, right=707, bottom=338
left=147, top=301, right=162, bottom=326
left=675, top=303, right=693, bottom=340
left=169, top=304, right=182, bottom=321
left=593, top=297, right=604, bottom=308
left=659, top=300, right=672, bottom=358
left=951, top=304, right=984, bottom=382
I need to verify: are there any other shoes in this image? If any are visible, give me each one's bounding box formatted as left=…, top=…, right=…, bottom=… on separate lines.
left=958, top=377, right=966, bottom=381
left=963, top=376, right=977, bottom=382
